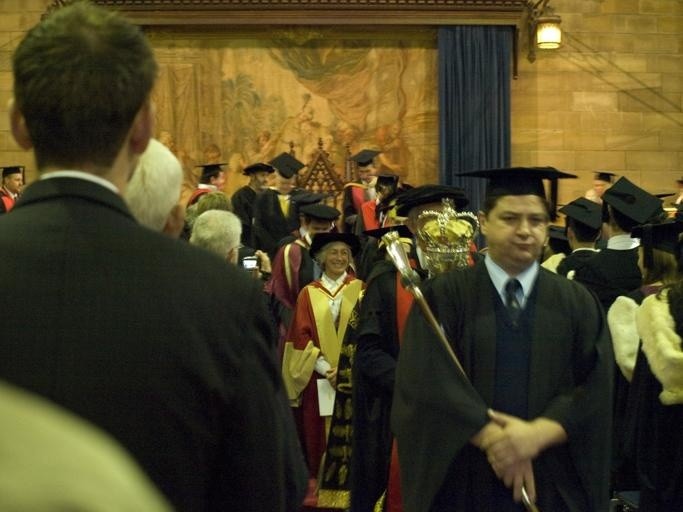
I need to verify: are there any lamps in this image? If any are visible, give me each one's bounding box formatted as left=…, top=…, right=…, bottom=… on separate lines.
left=531, top=15, right=563, bottom=54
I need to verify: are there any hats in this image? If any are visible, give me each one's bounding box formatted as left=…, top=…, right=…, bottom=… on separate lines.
left=591, top=170, right=615, bottom=182
left=361, top=224, right=413, bottom=239
left=298, top=203, right=341, bottom=222
left=630, top=221, right=683, bottom=254
left=599, top=176, right=664, bottom=225
left=558, top=196, right=602, bottom=231
left=287, top=192, right=324, bottom=204
left=241, top=162, right=275, bottom=176
left=194, top=163, right=229, bottom=170
left=309, top=232, right=361, bottom=257
left=348, top=149, right=402, bottom=185
left=395, top=181, right=471, bottom=213
left=0, top=166, right=26, bottom=175
left=268, top=151, right=304, bottom=178
left=453, top=165, right=580, bottom=196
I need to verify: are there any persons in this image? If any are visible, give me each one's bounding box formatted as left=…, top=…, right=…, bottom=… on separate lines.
left=1, top=164, right=24, bottom=214
left=0, top=0, right=313, bottom=511
left=159, top=104, right=409, bottom=189
left=118, top=135, right=183, bottom=241
left=0, top=375, right=176, bottom=512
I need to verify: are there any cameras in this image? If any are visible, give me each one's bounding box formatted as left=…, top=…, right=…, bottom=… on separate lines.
left=244, top=257, right=261, bottom=271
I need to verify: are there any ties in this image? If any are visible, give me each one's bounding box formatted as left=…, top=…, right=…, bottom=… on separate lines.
left=505, top=278, right=522, bottom=324
